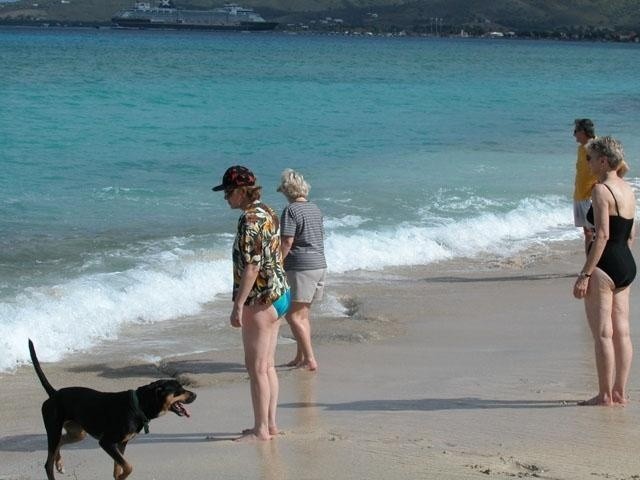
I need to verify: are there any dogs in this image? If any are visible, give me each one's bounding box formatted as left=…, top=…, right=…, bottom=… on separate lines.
left=28, top=337, right=197, bottom=480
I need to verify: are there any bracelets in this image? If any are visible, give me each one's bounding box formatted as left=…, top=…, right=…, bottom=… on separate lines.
left=578, top=272, right=592, bottom=278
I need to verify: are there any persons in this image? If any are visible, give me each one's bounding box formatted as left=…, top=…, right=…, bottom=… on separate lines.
left=276, top=168, right=326, bottom=371
left=573, top=118, right=630, bottom=262
left=573, top=134, right=637, bottom=406
left=211, top=164, right=291, bottom=444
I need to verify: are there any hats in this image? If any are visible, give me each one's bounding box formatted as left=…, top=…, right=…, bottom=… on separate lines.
left=211, top=165, right=256, bottom=191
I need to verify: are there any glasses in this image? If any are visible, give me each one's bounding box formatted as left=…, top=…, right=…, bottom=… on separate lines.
left=586, top=154, right=592, bottom=162
left=224, top=187, right=241, bottom=195
left=574, top=128, right=583, bottom=134
